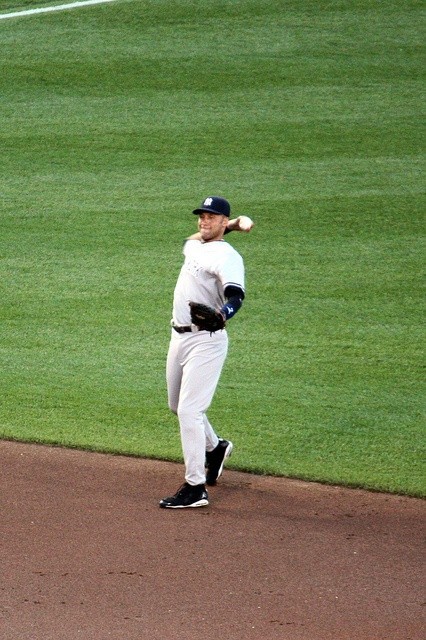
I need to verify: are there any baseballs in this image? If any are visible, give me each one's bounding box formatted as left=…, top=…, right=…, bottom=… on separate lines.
left=238, top=216, right=252, bottom=230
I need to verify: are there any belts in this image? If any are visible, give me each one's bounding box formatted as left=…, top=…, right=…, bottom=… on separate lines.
left=168, top=320, right=200, bottom=334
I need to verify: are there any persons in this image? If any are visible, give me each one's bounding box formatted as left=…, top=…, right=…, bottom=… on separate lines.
left=159, top=197, right=254, bottom=508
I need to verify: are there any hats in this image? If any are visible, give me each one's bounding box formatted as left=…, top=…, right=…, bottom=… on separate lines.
left=192, top=196, right=230, bottom=217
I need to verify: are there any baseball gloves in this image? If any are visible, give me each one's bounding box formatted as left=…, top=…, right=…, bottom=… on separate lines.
left=189, top=302, right=224, bottom=332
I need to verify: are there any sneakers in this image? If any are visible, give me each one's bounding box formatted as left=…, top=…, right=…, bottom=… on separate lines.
left=159, top=483, right=209, bottom=509
left=205, top=438, right=233, bottom=486
